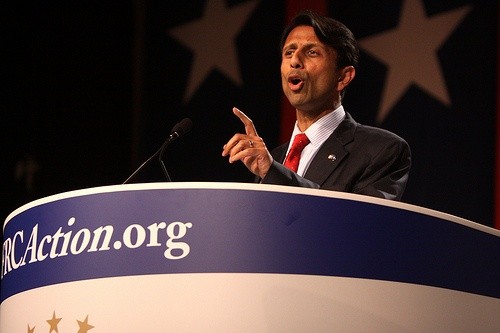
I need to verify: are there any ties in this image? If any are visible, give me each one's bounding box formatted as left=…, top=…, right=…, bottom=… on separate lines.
left=283, top=133, right=311, bottom=174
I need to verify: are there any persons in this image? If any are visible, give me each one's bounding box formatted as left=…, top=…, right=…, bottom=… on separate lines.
left=222, top=14, right=411, bottom=202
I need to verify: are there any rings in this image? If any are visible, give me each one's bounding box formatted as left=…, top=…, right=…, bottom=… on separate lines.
left=249, top=140, right=254, bottom=148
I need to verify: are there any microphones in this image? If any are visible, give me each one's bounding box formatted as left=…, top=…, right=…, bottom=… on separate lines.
left=158, top=123, right=178, bottom=182
left=122, top=118, right=191, bottom=184
